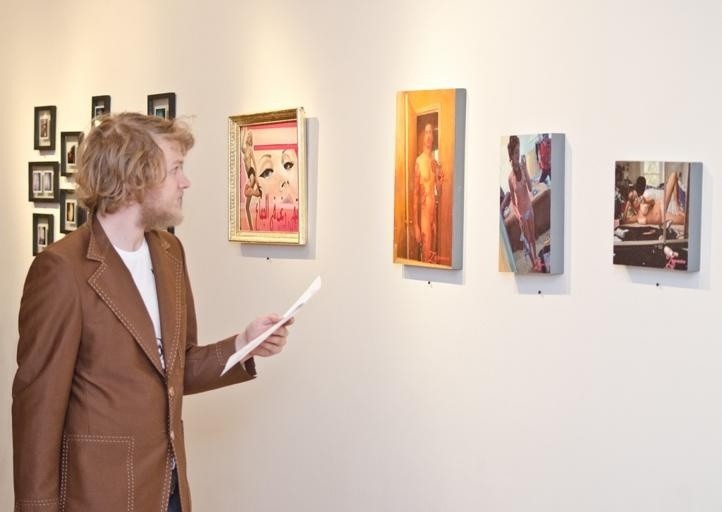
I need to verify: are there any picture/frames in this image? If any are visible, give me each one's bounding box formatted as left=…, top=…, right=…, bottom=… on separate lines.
left=225, top=105, right=310, bottom=247
left=25, top=87, right=179, bottom=260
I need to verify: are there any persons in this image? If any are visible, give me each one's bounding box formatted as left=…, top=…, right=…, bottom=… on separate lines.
left=624, top=172, right=686, bottom=225
left=414, top=121, right=440, bottom=262
left=501, top=134, right=552, bottom=272
left=241, top=126, right=263, bottom=232
left=11, top=111, right=293, bottom=512
left=253, top=149, right=299, bottom=210
